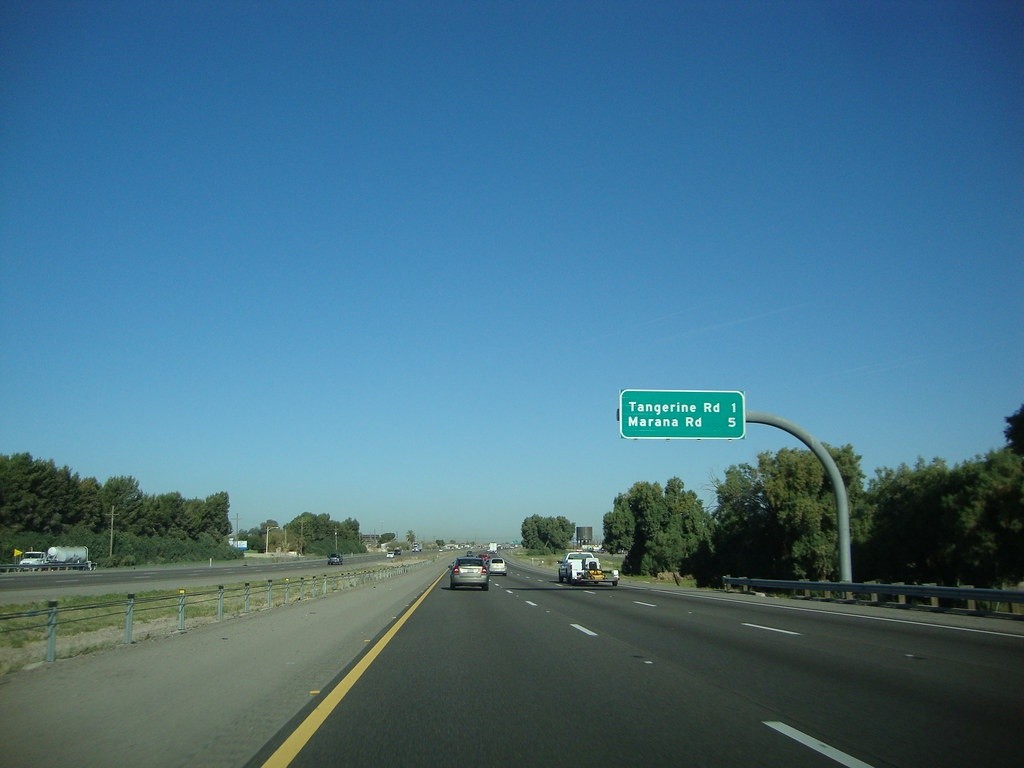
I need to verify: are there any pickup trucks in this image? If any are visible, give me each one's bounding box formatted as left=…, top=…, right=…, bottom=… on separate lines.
left=557, top=551, right=600, bottom=583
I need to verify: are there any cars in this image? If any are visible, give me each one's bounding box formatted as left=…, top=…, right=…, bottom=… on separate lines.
left=386, top=551, right=394, bottom=558
left=465, top=551, right=489, bottom=562
left=489, top=557, right=508, bottom=576
left=394, top=548, right=401, bottom=555
left=447, top=556, right=490, bottom=592
left=327, top=552, right=343, bottom=565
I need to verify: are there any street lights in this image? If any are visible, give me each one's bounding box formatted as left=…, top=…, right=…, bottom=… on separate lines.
left=266, top=526, right=279, bottom=554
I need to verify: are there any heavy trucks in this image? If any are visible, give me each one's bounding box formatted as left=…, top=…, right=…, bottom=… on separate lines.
left=18, top=545, right=98, bottom=571
